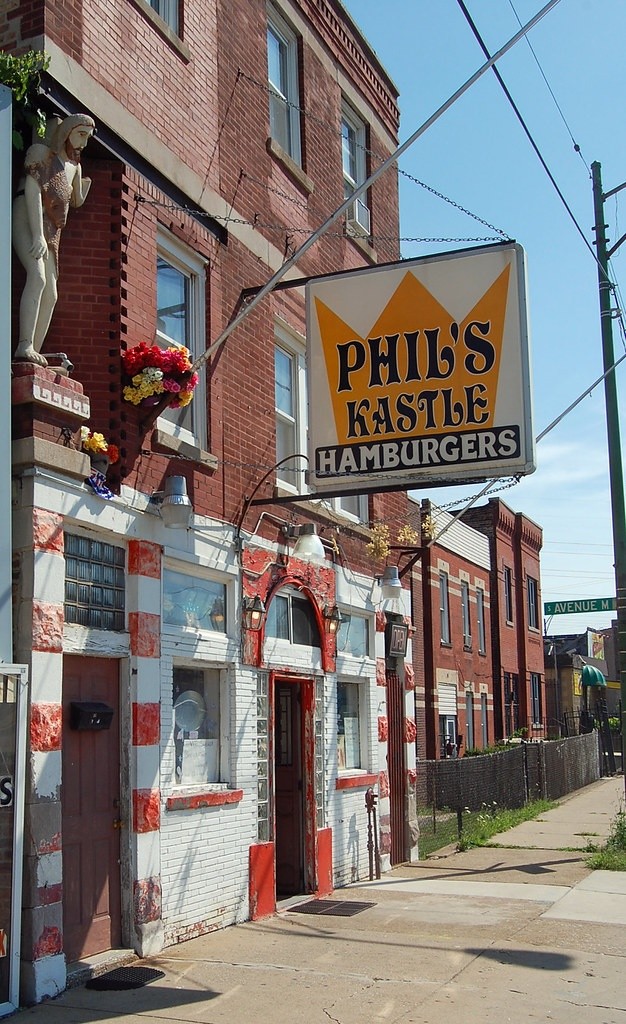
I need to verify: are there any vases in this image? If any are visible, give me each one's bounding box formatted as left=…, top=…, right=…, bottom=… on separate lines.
left=81, top=449, right=110, bottom=475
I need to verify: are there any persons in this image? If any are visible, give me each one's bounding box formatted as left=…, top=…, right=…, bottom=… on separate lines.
left=13, top=114, right=96, bottom=368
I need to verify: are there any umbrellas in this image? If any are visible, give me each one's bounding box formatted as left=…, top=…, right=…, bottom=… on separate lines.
left=579, top=664, right=607, bottom=734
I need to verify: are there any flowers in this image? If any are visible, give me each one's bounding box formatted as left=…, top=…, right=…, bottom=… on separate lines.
left=121, top=341, right=200, bottom=410
left=80, top=425, right=120, bottom=466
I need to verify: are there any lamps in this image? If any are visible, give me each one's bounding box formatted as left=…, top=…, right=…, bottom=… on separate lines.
left=374, top=566, right=402, bottom=599
left=152, top=474, right=192, bottom=530
left=241, top=593, right=267, bottom=632
left=282, top=522, right=326, bottom=562
